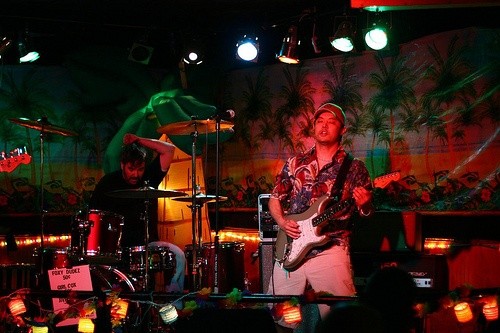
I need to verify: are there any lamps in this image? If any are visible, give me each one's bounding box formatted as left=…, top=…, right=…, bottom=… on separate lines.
left=183, top=31, right=207, bottom=65
left=328, top=16, right=361, bottom=54
left=283, top=306, right=302, bottom=324
left=11, top=29, right=41, bottom=64
left=31, top=326, right=49, bottom=333
left=454, top=302, right=473, bottom=323
left=363, top=16, right=393, bottom=51
left=127, top=29, right=155, bottom=65
left=158, top=304, right=179, bottom=326
left=110, top=300, right=129, bottom=319
left=7, top=295, right=26, bottom=316
left=275, top=22, right=307, bottom=66
left=235, top=22, right=272, bottom=64
left=483, top=302, right=499, bottom=320
left=78, top=315, right=95, bottom=333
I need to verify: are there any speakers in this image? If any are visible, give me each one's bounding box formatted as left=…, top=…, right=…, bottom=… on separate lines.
left=257, top=242, right=277, bottom=294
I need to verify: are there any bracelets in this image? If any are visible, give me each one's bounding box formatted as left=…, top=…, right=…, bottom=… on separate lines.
left=361, top=209, right=372, bottom=216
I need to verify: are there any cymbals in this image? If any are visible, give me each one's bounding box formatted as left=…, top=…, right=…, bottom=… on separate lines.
left=6, top=117, right=80, bottom=137
left=103, top=189, right=189, bottom=199
left=170, top=193, right=229, bottom=204
left=157, top=120, right=234, bottom=135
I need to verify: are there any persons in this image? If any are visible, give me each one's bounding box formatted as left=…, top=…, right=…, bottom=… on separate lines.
left=265, top=102, right=372, bottom=329
left=86, top=133, right=176, bottom=333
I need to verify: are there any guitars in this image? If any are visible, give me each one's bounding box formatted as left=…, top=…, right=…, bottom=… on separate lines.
left=274, top=170, right=400, bottom=268
left=0, top=145, right=31, bottom=173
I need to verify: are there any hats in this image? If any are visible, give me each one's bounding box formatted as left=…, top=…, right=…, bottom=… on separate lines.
left=314, top=103, right=346, bottom=124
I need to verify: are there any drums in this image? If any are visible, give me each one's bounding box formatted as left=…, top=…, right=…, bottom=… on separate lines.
left=125, top=246, right=171, bottom=293
left=68, top=209, right=125, bottom=263
left=31, top=266, right=140, bottom=333
left=185, top=241, right=249, bottom=295
left=34, top=247, right=84, bottom=283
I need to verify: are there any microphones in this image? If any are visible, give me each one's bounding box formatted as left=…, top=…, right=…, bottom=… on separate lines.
left=208, top=110, right=235, bottom=121
